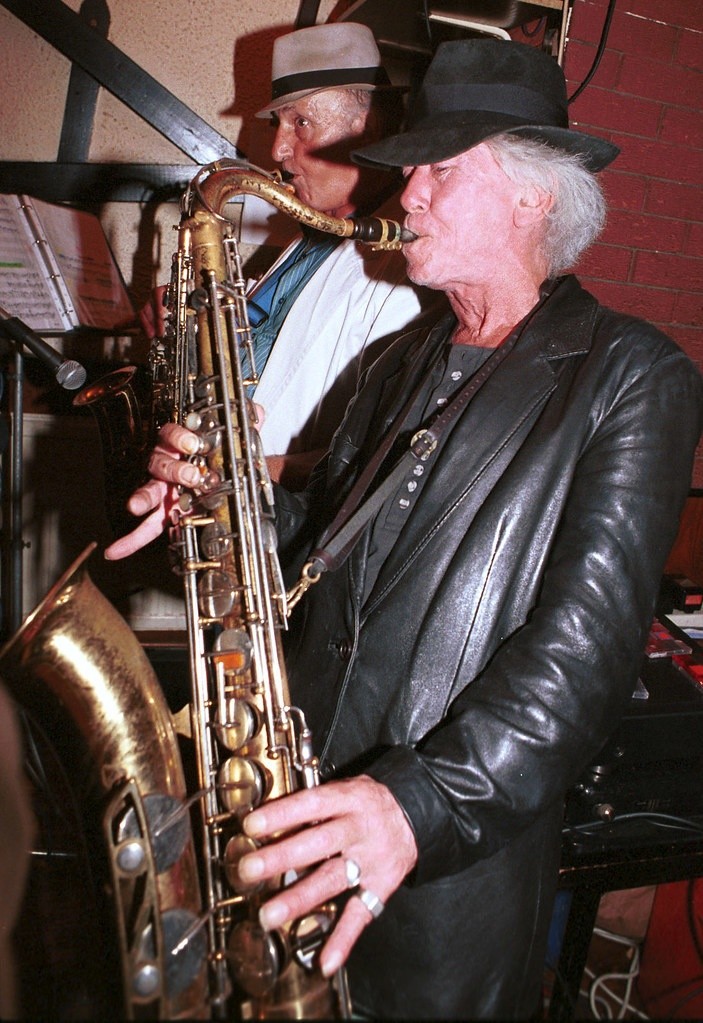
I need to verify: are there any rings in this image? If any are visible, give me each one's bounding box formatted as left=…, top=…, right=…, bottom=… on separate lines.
left=340, top=855, right=359, bottom=889
left=355, top=887, right=385, bottom=918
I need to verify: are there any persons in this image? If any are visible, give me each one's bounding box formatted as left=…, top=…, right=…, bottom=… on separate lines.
left=101, top=38, right=703, bottom=1023
left=139, top=23, right=448, bottom=460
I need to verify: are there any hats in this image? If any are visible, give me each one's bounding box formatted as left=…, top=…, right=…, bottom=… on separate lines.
left=248, top=20, right=409, bottom=115
left=352, top=38, right=620, bottom=173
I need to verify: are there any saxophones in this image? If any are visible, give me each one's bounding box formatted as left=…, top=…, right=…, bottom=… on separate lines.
left=2, top=168, right=416, bottom=1023
left=73, top=161, right=290, bottom=585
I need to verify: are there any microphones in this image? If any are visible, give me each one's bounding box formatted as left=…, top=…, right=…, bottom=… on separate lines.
left=0, top=306, right=88, bottom=390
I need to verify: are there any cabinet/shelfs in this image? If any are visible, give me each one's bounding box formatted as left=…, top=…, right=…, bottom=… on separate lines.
left=560, top=654, right=703, bottom=1023
